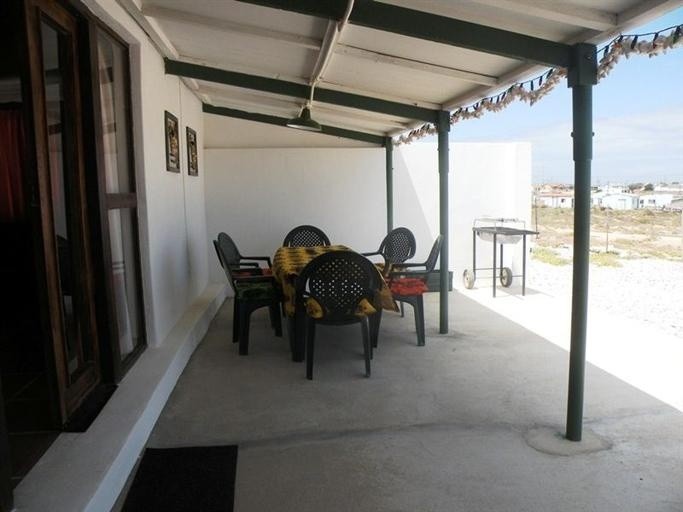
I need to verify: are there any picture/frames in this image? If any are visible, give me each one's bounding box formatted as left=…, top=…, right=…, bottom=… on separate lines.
left=186, top=127, right=198, bottom=176
left=164, top=110, right=180, bottom=173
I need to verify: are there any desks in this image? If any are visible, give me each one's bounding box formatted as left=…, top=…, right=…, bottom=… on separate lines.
left=271, top=245, right=400, bottom=361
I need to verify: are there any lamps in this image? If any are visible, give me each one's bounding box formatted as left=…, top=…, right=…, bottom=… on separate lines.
left=286, top=81, right=323, bottom=133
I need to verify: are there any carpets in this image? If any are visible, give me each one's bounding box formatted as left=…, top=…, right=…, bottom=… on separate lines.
left=118, top=445, right=238, bottom=511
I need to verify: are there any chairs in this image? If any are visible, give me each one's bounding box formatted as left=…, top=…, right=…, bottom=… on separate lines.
left=295, top=250, right=382, bottom=380
left=359, top=228, right=417, bottom=318
left=218, top=232, right=286, bottom=319
left=284, top=226, right=331, bottom=246
left=213, top=240, right=294, bottom=356
left=370, top=235, right=445, bottom=347
